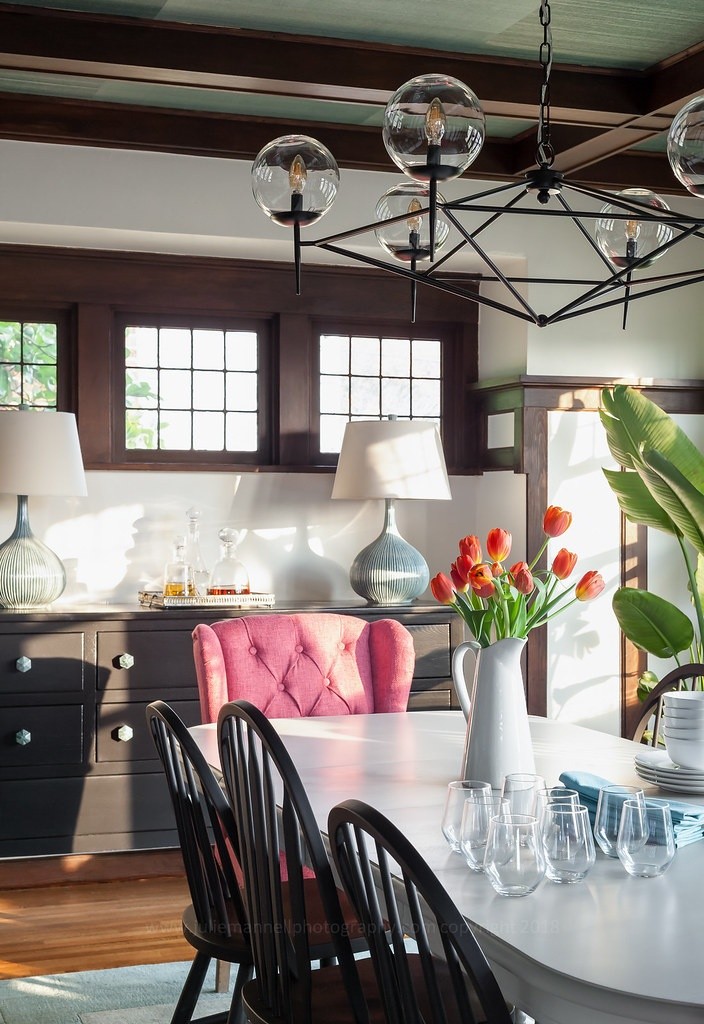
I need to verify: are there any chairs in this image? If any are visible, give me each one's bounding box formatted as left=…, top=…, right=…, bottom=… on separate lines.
left=216, top=698, right=516, bottom=1024
left=190, top=611, right=419, bottom=990
left=627, top=663, right=704, bottom=751
left=325, top=796, right=536, bottom=1024
left=146, top=699, right=408, bottom=1024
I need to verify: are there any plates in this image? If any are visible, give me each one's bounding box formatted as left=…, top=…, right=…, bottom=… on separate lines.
left=634, top=750, right=704, bottom=795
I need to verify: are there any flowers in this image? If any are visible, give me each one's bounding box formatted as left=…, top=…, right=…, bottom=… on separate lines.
left=430, top=504, right=605, bottom=648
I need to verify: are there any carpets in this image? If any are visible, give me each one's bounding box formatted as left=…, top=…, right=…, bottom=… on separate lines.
left=0, top=936, right=419, bottom=1024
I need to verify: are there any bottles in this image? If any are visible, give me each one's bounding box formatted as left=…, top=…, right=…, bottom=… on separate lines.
left=182, top=506, right=210, bottom=595
left=208, top=527, right=250, bottom=595
left=163, top=536, right=196, bottom=598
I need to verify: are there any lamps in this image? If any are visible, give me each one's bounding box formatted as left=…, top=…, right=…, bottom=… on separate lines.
left=250, top=1, right=704, bottom=330
left=329, top=412, right=454, bottom=605
left=0, top=403, right=89, bottom=608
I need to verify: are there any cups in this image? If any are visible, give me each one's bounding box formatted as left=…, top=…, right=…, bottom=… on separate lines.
left=458, top=797, right=514, bottom=873
left=483, top=814, right=547, bottom=897
left=498, top=773, right=549, bottom=847
left=536, top=803, right=596, bottom=884
left=529, top=788, right=585, bottom=861
left=616, top=800, right=677, bottom=878
left=442, top=780, right=494, bottom=854
left=593, top=785, right=649, bottom=859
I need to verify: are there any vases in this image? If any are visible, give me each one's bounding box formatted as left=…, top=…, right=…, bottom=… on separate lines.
left=451, top=637, right=537, bottom=792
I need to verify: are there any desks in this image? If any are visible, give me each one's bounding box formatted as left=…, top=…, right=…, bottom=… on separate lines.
left=163, top=709, right=704, bottom=1024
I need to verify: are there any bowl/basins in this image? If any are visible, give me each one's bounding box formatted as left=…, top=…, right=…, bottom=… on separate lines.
left=663, top=689, right=704, bottom=770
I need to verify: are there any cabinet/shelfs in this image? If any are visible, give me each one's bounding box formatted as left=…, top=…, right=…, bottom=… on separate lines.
left=464, top=374, right=704, bottom=750
left=0, top=599, right=530, bottom=894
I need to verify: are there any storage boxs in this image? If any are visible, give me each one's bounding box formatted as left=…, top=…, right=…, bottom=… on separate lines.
left=138, top=590, right=276, bottom=610
